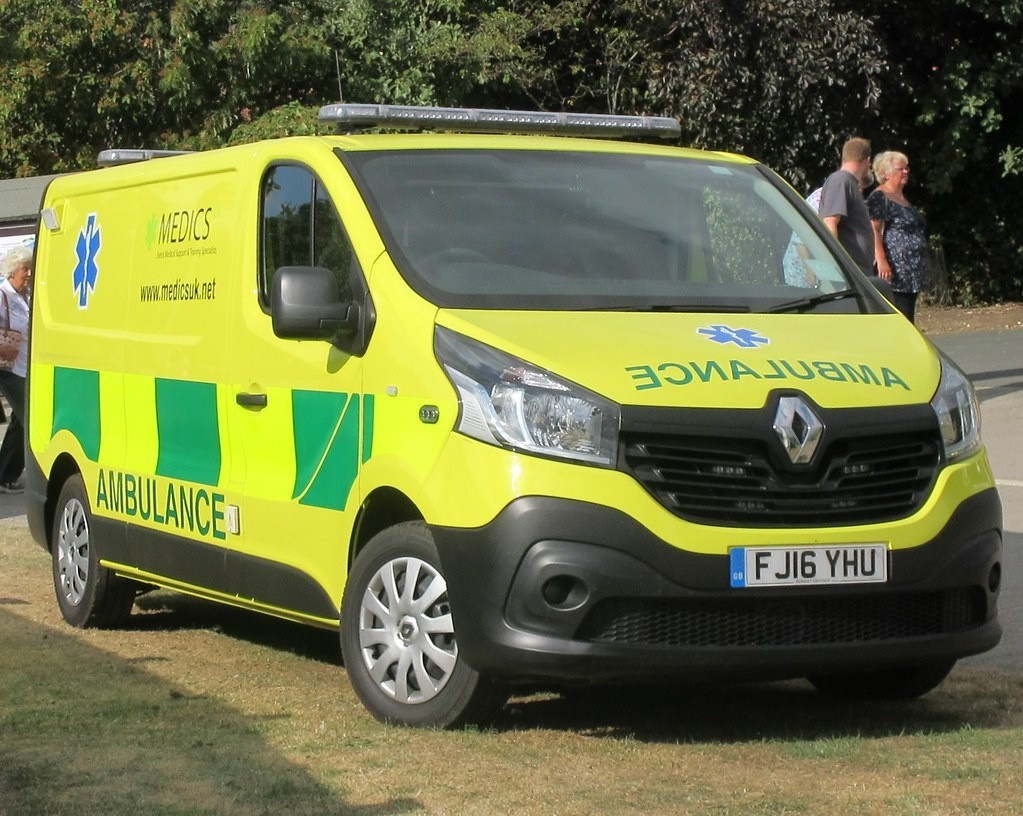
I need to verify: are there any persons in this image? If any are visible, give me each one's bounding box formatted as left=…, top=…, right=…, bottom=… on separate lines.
left=782, top=169, right=874, bottom=289
left=0, top=246, right=35, bottom=493
left=867, top=152, right=933, bottom=325
left=818, top=139, right=875, bottom=278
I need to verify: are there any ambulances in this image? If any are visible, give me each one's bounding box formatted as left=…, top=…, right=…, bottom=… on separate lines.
left=19, top=103, right=1006, bottom=729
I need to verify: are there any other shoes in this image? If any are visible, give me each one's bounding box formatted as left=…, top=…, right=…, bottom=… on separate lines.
left=0, top=481, right=25, bottom=494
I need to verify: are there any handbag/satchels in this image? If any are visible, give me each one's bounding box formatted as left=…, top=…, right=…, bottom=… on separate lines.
left=873, top=242, right=898, bottom=279
left=0, top=288, right=22, bottom=369
